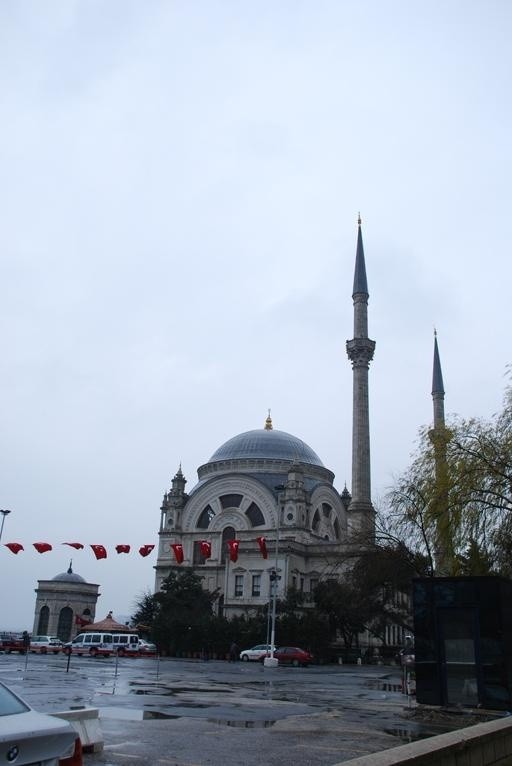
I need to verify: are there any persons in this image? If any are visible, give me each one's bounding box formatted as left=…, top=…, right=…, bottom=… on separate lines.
left=227, top=642, right=239, bottom=664
left=19, top=631, right=31, bottom=654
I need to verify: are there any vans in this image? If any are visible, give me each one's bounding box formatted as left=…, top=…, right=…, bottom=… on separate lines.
left=0, top=631, right=157, bottom=658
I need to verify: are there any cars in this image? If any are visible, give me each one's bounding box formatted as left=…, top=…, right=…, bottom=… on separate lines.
left=239, top=644, right=315, bottom=667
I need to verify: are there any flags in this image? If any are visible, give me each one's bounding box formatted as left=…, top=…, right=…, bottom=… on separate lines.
left=226, top=539, right=240, bottom=563
left=139, top=544, right=156, bottom=557
left=75, top=615, right=91, bottom=625
left=33, top=542, right=54, bottom=554
left=62, top=542, right=84, bottom=550
left=115, top=545, right=131, bottom=554
left=90, top=544, right=108, bottom=560
left=256, top=536, right=268, bottom=561
left=170, top=543, right=185, bottom=564
left=4, top=542, right=26, bottom=555
left=198, top=541, right=212, bottom=558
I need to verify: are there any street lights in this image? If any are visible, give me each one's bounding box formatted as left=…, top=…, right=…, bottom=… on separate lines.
left=263, top=484, right=286, bottom=668
